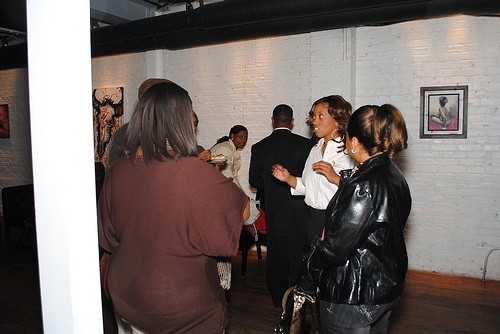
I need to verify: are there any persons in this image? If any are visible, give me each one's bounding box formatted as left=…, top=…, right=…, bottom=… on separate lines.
left=98, top=83, right=251, bottom=334
left=432, top=96, right=451, bottom=130
left=248, top=103, right=314, bottom=310
left=270, top=94, right=358, bottom=238
left=108, top=78, right=229, bottom=170
left=308, top=103, right=412, bottom=334
left=205, top=125, right=248, bottom=192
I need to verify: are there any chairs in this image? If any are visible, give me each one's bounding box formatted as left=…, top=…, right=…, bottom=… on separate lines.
left=235, top=230, right=262, bottom=280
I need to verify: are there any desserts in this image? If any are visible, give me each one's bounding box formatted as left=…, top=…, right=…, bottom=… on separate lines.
left=210, top=154, right=226, bottom=159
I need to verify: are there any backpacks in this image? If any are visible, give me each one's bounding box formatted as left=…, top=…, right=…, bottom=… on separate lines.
left=274, top=284, right=321, bottom=334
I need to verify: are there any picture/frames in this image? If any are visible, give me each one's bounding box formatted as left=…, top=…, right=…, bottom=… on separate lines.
left=419, top=85, right=469, bottom=139
